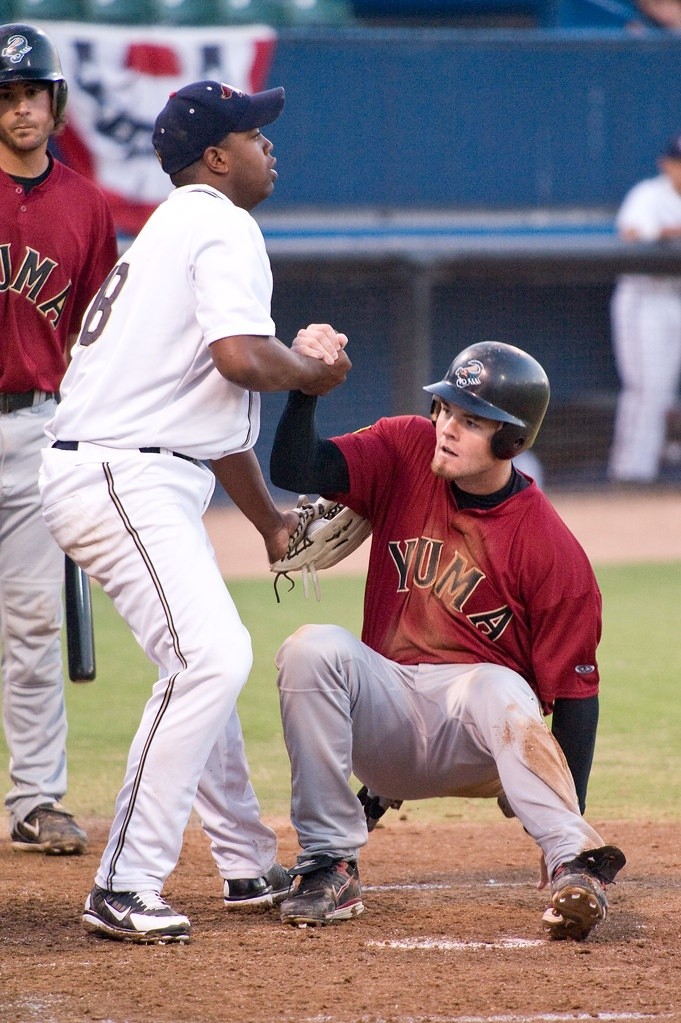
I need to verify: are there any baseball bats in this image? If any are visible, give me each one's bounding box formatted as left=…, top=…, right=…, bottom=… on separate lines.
left=64, top=554, right=99, bottom=684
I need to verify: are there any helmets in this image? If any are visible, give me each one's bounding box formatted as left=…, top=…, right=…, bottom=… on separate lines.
left=0, top=23, right=67, bottom=117
left=422, top=341, right=550, bottom=461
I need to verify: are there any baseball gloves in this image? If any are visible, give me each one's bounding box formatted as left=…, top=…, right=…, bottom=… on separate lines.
left=269, top=495, right=373, bottom=575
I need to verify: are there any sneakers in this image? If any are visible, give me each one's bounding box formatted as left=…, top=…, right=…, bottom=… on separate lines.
left=222, top=860, right=295, bottom=913
left=82, top=883, right=190, bottom=946
left=279, top=856, right=364, bottom=928
left=541, top=846, right=626, bottom=942
left=10, top=801, right=88, bottom=854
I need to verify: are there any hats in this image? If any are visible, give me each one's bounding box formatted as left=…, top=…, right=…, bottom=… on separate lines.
left=152, top=81, right=285, bottom=174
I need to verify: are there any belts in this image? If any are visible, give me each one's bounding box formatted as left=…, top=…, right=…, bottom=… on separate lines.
left=0, top=389, right=61, bottom=414
left=51, top=440, right=197, bottom=462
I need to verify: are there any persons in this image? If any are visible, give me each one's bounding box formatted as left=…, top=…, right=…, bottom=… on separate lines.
left=268, top=324, right=628, bottom=940
left=546, top=0, right=681, bottom=28
left=0, top=23, right=119, bottom=854
left=609, top=136, right=681, bottom=483
left=38, top=83, right=347, bottom=941
